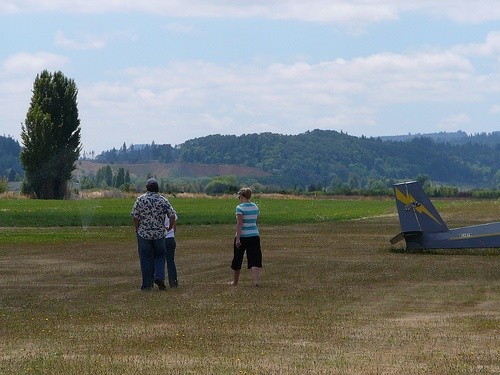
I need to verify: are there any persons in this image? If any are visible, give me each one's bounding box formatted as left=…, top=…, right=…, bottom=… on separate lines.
left=154, top=200, right=179, bottom=287
left=228, top=188, right=263, bottom=286
left=131, top=178, right=176, bottom=291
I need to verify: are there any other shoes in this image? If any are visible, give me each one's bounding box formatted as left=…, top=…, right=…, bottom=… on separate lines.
left=142, top=285, right=153, bottom=292
left=155, top=277, right=166, bottom=290
left=170, top=282, right=178, bottom=289
left=228, top=282, right=239, bottom=286
